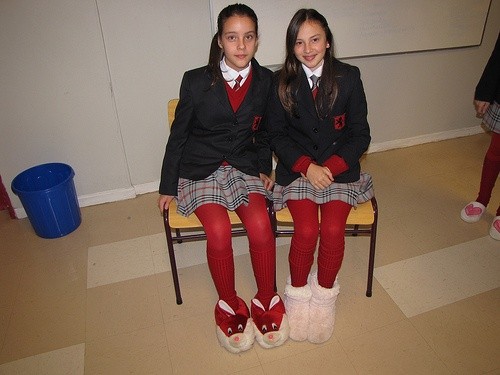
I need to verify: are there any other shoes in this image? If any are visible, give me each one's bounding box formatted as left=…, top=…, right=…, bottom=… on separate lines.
left=215, top=296, right=255, bottom=353
left=251, top=293, right=289, bottom=349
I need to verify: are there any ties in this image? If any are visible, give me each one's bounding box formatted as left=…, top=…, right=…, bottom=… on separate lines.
left=233, top=75, right=243, bottom=92
left=310, top=74, right=319, bottom=103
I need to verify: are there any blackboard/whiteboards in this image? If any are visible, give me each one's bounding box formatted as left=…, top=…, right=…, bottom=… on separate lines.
left=209, top=0, right=493, bottom=69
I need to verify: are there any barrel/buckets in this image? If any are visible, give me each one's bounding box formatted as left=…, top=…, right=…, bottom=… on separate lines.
left=11, top=162, right=82, bottom=239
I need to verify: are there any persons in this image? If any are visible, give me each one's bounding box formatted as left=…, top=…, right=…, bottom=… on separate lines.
left=264, top=9, right=374, bottom=345
left=459, top=32, right=500, bottom=241
left=158, top=3, right=291, bottom=355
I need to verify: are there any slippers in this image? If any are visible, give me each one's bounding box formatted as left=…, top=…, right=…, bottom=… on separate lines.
left=490, top=216, right=500, bottom=240
left=461, top=194, right=489, bottom=224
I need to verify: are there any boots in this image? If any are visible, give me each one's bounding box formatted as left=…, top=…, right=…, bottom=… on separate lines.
left=284, top=273, right=340, bottom=344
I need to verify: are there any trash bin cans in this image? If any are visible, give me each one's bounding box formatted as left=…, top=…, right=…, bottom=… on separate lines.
left=11, top=162, right=81, bottom=239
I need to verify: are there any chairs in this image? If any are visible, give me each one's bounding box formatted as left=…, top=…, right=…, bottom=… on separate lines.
left=162, top=99, right=379, bottom=305
